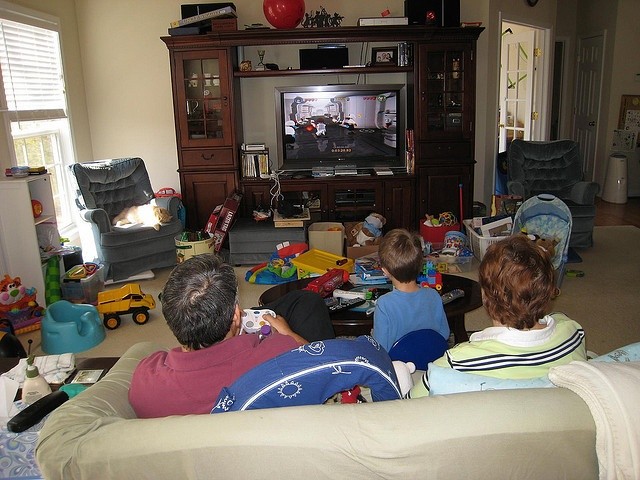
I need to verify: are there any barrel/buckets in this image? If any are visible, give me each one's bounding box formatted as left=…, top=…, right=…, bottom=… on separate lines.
left=173, top=235, right=215, bottom=265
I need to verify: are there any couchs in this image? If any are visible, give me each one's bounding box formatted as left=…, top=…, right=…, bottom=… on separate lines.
left=65, top=154, right=189, bottom=282
left=35, top=339, right=640, bottom=480
left=226, top=209, right=310, bottom=268
left=500, top=139, right=603, bottom=253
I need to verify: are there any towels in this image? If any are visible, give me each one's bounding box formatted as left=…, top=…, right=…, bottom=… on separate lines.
left=548, top=356, right=639, bottom=480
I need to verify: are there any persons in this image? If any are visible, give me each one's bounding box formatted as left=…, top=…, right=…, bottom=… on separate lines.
left=381, top=53, right=390, bottom=62
left=386, top=52, right=392, bottom=62
left=371, top=229, right=452, bottom=353
left=126, top=252, right=311, bottom=419
left=377, top=56, right=381, bottom=62
left=401, top=234, right=586, bottom=398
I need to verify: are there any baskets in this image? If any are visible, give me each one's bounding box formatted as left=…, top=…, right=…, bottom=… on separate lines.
left=462, top=219, right=511, bottom=261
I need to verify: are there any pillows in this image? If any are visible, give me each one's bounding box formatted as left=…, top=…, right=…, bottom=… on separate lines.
left=425, top=339, right=640, bottom=399
left=213, top=334, right=405, bottom=411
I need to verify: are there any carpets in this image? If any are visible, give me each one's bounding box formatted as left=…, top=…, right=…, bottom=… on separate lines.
left=2, top=221, right=640, bottom=357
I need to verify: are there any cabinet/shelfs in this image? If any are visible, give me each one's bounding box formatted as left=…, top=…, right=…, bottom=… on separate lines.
left=233, top=24, right=415, bottom=78
left=415, top=24, right=485, bottom=235
left=329, top=175, right=384, bottom=222
left=160, top=27, right=244, bottom=243
left=271, top=173, right=328, bottom=222
left=0, top=171, right=64, bottom=310
left=381, top=174, right=419, bottom=237
left=240, top=175, right=270, bottom=220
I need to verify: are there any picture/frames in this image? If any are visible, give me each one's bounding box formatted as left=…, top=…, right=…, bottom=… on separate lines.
left=372, top=45, right=399, bottom=67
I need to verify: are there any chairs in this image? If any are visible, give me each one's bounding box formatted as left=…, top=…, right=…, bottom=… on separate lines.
left=508, top=193, right=574, bottom=300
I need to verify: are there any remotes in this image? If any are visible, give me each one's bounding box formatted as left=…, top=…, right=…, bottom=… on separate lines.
left=328, top=298, right=366, bottom=314
left=440, top=289, right=465, bottom=306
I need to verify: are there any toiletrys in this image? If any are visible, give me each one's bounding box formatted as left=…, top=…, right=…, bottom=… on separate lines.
left=22, top=353, right=51, bottom=407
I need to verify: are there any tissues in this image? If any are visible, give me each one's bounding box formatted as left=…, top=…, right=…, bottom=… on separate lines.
left=0, top=376, right=50, bottom=480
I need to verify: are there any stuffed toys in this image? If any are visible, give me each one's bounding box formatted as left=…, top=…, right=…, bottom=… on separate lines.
left=350, top=212, right=387, bottom=248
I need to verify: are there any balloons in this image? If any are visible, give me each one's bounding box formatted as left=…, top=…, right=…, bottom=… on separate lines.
left=261, top=0, right=306, bottom=29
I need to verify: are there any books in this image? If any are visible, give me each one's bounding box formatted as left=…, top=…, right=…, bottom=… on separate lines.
left=170, top=6, right=238, bottom=28
left=4, top=166, right=48, bottom=176
left=354, top=261, right=389, bottom=281
left=349, top=273, right=386, bottom=287
left=357, top=17, right=409, bottom=27
left=273, top=208, right=311, bottom=227
left=242, top=153, right=268, bottom=177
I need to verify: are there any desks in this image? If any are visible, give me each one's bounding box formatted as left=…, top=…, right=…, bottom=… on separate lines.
left=11, top=356, right=118, bottom=428
left=257, top=271, right=482, bottom=350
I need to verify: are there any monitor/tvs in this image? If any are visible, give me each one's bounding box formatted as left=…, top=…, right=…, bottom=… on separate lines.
left=274, top=83, right=408, bottom=178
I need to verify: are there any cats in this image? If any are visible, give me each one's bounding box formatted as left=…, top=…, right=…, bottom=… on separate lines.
left=111, top=203, right=174, bottom=232
